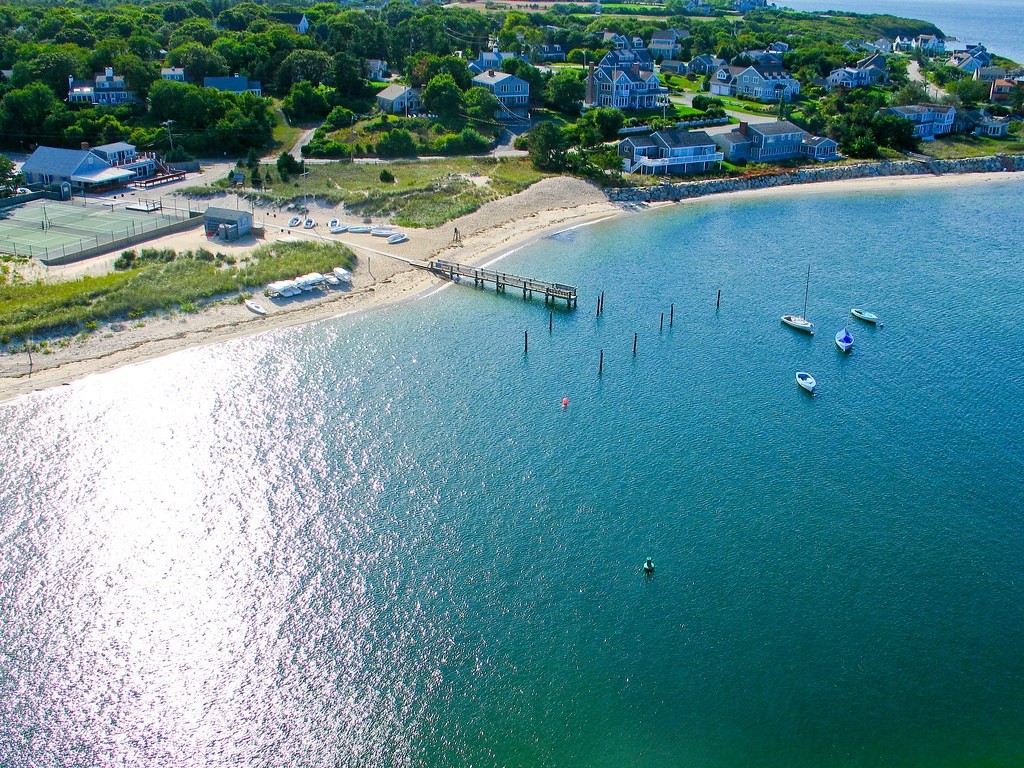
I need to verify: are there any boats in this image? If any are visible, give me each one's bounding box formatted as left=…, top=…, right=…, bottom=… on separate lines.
left=304, top=217, right=316, bottom=229
left=795, top=372, right=817, bottom=391
left=330, top=225, right=350, bottom=234
left=348, top=225, right=374, bottom=233
left=834, top=327, right=854, bottom=352
left=329, top=217, right=340, bottom=229
left=386, top=233, right=408, bottom=244
left=849, top=307, right=879, bottom=323
left=370, top=229, right=396, bottom=236
left=288, top=215, right=302, bottom=227
left=267, top=267, right=353, bottom=298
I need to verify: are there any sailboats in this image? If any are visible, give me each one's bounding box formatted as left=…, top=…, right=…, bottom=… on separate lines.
left=780, top=264, right=815, bottom=332
